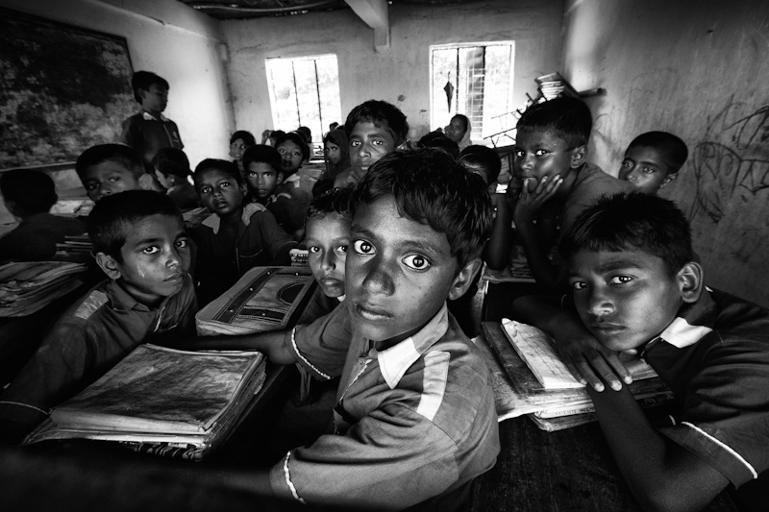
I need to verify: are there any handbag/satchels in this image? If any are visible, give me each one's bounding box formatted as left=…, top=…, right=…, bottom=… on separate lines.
left=193, top=265, right=327, bottom=335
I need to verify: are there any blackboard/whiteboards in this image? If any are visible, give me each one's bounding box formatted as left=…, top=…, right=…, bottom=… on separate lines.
left=0, top=6, right=142, bottom=175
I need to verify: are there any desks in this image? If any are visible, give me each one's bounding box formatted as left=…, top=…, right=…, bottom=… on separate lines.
left=0, top=250, right=340, bottom=512
left=0, top=201, right=231, bottom=400
left=442, top=231, right=734, bottom=512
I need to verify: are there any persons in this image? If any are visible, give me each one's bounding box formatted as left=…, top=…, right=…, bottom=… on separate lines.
left=0, top=70, right=769, bottom=511
